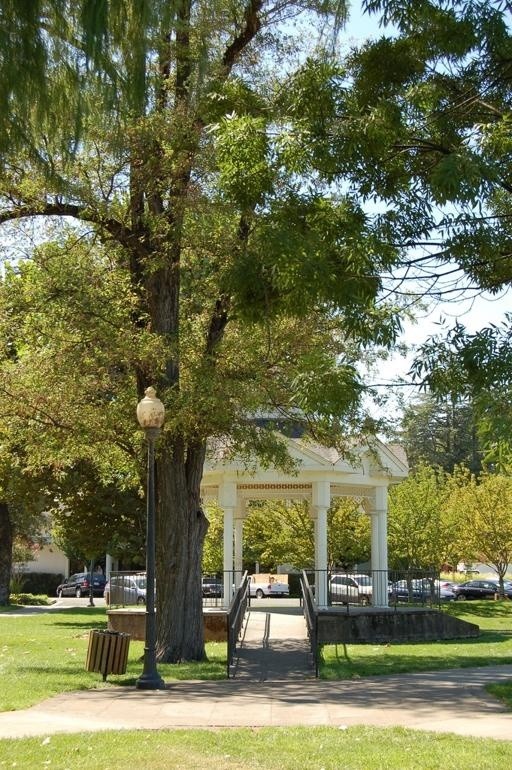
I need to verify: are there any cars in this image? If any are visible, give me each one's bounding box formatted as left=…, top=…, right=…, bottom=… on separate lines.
left=56, top=572, right=105, bottom=598
left=103, top=575, right=156, bottom=604
left=388, top=574, right=512, bottom=604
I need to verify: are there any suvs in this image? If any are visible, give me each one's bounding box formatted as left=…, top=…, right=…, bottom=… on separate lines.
left=328, top=574, right=392, bottom=606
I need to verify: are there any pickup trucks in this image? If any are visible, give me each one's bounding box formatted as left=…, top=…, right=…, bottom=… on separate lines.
left=201, top=578, right=222, bottom=597
left=232, top=575, right=290, bottom=598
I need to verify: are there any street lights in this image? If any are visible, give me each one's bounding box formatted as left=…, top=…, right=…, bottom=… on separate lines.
left=136, top=384, right=166, bottom=690
